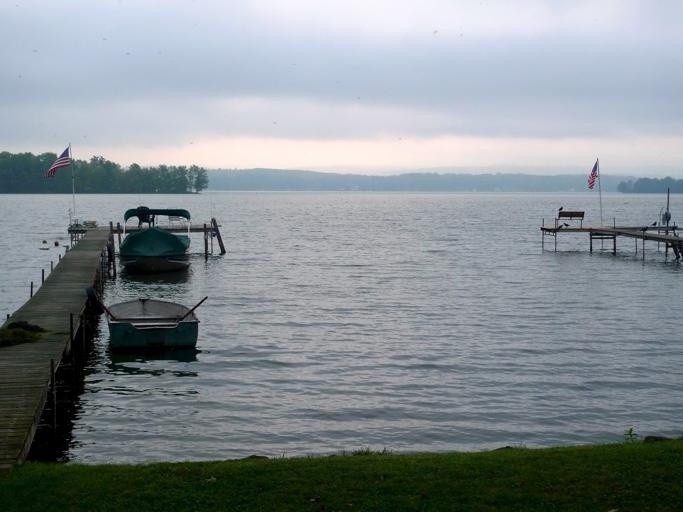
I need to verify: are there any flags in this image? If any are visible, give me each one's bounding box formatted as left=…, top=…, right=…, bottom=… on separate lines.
left=47, top=145, right=71, bottom=178
left=587, top=161, right=597, bottom=190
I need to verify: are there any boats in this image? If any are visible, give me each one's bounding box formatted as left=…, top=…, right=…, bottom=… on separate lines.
left=106, top=339, right=199, bottom=380
left=118, top=264, right=189, bottom=287
left=105, top=297, right=202, bottom=351
left=116, top=206, right=193, bottom=273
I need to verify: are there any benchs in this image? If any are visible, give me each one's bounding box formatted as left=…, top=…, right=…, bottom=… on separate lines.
left=556, top=210, right=585, bottom=229
